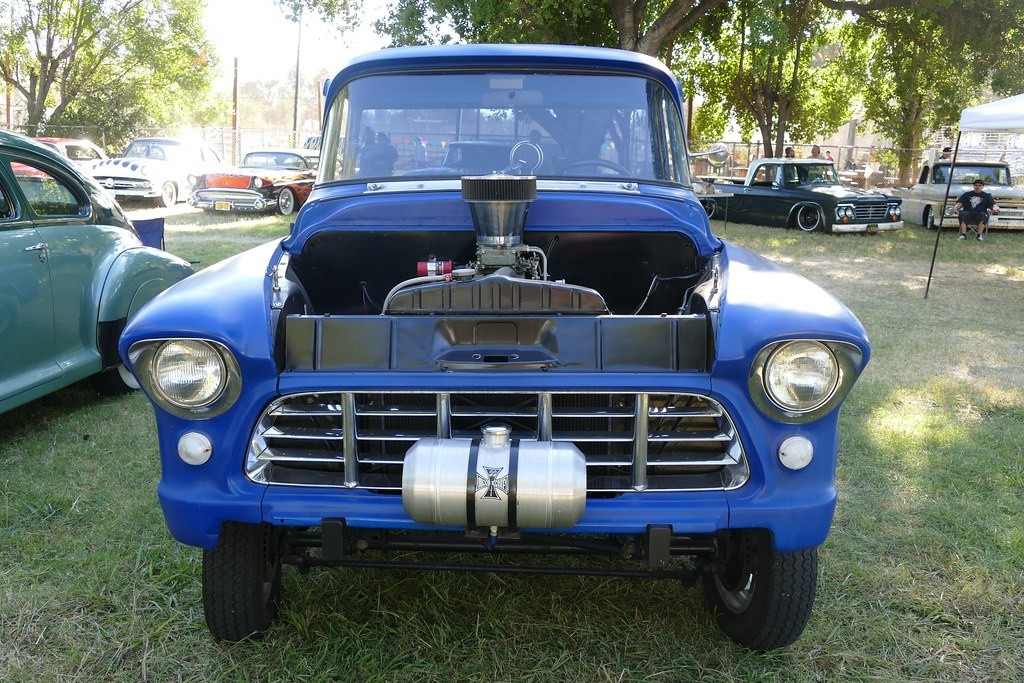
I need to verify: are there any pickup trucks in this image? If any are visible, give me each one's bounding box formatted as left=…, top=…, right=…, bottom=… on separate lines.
left=691, top=157, right=903, bottom=234
left=891, top=160, right=1024, bottom=233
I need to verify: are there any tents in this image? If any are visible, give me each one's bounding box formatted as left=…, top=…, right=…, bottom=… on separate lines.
left=925, top=94, right=1024, bottom=300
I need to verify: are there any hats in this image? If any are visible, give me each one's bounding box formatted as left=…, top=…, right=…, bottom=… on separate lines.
left=973, top=179, right=984, bottom=186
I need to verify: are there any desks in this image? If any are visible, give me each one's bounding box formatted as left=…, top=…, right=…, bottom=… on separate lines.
left=698, top=192, right=734, bottom=230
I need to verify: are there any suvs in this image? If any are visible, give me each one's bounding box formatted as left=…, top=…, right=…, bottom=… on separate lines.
left=89, top=137, right=224, bottom=210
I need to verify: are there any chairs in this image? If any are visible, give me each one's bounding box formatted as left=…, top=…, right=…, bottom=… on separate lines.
left=132, top=217, right=165, bottom=251
left=956, top=206, right=998, bottom=240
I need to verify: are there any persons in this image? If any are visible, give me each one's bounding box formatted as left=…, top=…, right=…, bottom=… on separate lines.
left=950, top=179, right=1000, bottom=241
left=417, top=137, right=427, bottom=169
left=776, top=147, right=796, bottom=185
left=360, top=131, right=397, bottom=174
left=826, top=151, right=834, bottom=162
left=805, top=145, right=826, bottom=182
left=935, top=147, right=953, bottom=183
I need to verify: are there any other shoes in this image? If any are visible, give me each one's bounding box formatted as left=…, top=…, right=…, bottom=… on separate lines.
left=957, top=234, right=966, bottom=240
left=977, top=234, right=983, bottom=240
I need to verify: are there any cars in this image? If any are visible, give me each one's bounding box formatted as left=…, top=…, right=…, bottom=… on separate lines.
left=189, top=146, right=343, bottom=218
left=118, top=42, right=874, bottom=652
left=0, top=125, right=195, bottom=420
left=10, top=136, right=110, bottom=180
